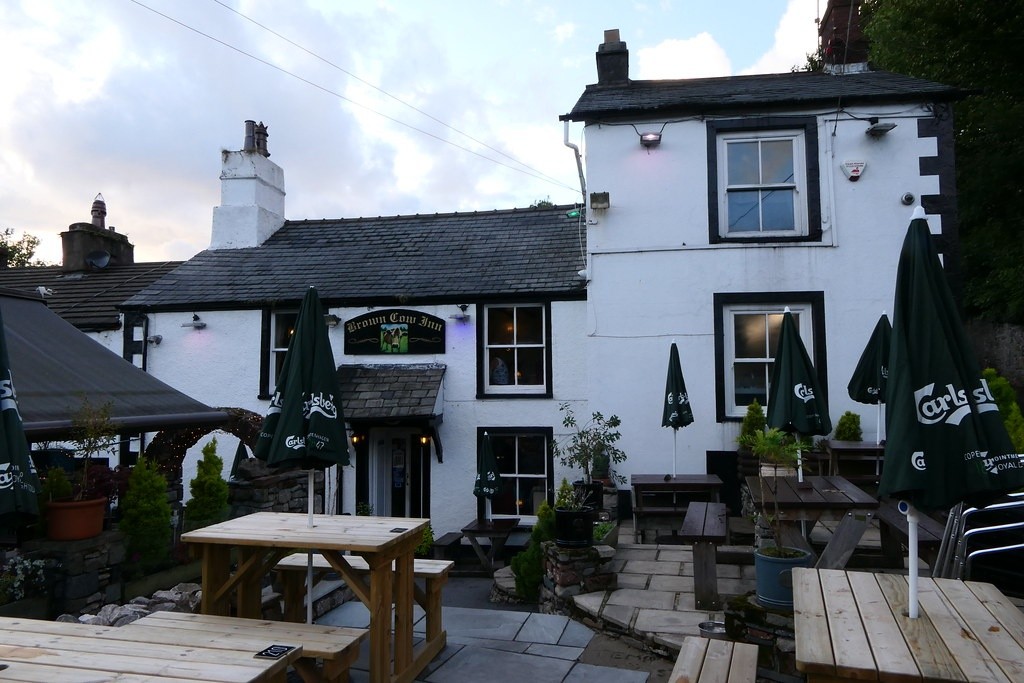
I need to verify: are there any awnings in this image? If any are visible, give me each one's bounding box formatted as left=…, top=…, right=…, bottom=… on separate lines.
left=0, top=289, right=228, bottom=433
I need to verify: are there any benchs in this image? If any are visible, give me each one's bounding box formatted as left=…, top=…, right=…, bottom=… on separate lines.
left=677, top=502, right=757, bottom=612
left=430, top=532, right=473, bottom=580
left=120, top=610, right=371, bottom=683
left=856, top=496, right=948, bottom=578
left=835, top=473, right=881, bottom=486
left=666, top=636, right=759, bottom=683
left=632, top=507, right=732, bottom=544
left=275, top=553, right=455, bottom=671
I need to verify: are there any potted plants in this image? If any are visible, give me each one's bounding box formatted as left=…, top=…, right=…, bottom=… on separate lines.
left=550, top=401, right=628, bottom=513
left=733, top=424, right=812, bottom=615
left=733, top=397, right=767, bottom=499
left=72, top=463, right=132, bottom=532
left=591, top=444, right=612, bottom=486
left=554, top=490, right=596, bottom=550
left=759, top=436, right=811, bottom=476
left=45, top=390, right=127, bottom=541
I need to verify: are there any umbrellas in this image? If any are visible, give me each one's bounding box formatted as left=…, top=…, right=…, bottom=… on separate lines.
left=849, top=313, right=893, bottom=474
left=662, top=342, right=694, bottom=507
left=230, top=438, right=250, bottom=477
left=256, top=283, right=351, bottom=624
left=474, top=431, right=502, bottom=566
left=766, top=306, right=833, bottom=539
left=0, top=315, right=44, bottom=519
left=878, top=206, right=1023, bottom=618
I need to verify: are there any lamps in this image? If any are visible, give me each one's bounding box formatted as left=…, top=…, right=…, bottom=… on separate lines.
left=589, top=191, right=610, bottom=210
left=865, top=122, right=898, bottom=136
left=418, top=433, right=430, bottom=457
left=640, top=131, right=662, bottom=148
left=324, top=314, right=342, bottom=326
left=351, top=431, right=361, bottom=451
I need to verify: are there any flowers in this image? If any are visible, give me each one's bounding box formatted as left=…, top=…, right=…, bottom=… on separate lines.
left=0, top=556, right=50, bottom=607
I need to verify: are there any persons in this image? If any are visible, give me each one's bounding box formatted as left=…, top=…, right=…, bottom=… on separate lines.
left=490, top=348, right=538, bottom=385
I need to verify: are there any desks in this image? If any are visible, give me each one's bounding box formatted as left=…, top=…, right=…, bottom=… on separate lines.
left=744, top=475, right=881, bottom=570
left=460, top=518, right=520, bottom=579
left=824, top=439, right=885, bottom=476
left=630, top=474, right=724, bottom=545
left=0, top=616, right=303, bottom=683
left=180, top=511, right=431, bottom=682
left=791, top=567, right=1024, bottom=683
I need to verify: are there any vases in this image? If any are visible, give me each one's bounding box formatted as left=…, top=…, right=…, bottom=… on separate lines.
left=0, top=596, right=48, bottom=620
